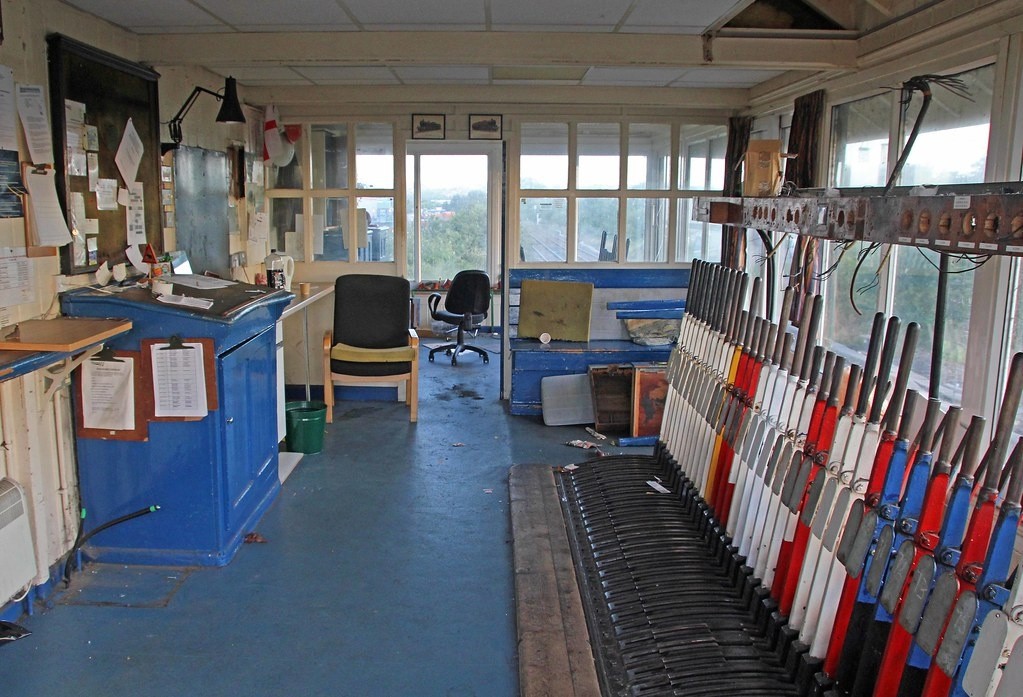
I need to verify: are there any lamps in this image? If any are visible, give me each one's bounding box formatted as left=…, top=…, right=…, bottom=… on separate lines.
left=168, top=75, right=247, bottom=144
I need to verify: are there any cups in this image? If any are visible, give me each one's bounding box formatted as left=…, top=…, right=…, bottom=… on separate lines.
left=299, top=282, right=311, bottom=296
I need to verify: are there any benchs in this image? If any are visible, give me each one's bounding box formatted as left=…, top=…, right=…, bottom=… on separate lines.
left=507, top=267, right=692, bottom=417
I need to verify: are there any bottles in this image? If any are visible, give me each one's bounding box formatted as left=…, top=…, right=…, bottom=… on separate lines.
left=265, top=248, right=286, bottom=291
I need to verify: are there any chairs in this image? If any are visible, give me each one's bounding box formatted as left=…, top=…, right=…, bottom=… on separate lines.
left=427, top=270, right=493, bottom=366
left=322, top=274, right=420, bottom=425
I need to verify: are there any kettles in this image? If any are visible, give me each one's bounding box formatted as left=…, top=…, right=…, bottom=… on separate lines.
left=280, top=255, right=295, bottom=292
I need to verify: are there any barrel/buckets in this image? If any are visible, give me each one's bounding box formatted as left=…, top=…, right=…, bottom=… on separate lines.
left=285, top=399, right=329, bottom=456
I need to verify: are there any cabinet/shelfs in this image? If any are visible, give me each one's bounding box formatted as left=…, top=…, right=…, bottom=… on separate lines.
left=61, top=270, right=297, bottom=569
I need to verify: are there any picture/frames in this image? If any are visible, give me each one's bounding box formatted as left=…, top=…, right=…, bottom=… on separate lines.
left=469, top=114, right=503, bottom=140
left=411, top=113, right=446, bottom=140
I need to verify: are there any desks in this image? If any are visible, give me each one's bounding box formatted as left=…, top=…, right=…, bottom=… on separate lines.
left=282, top=281, right=336, bottom=402
